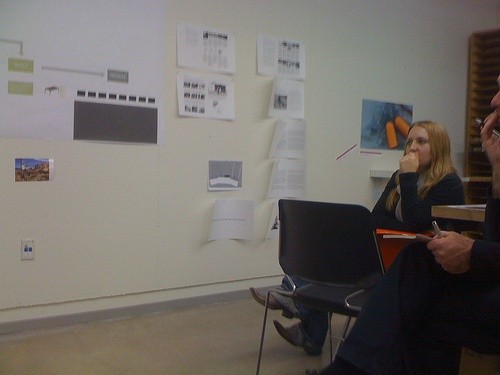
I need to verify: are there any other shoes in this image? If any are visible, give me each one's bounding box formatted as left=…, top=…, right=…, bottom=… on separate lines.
left=252, top=287, right=294, bottom=318
left=273, top=319, right=322, bottom=355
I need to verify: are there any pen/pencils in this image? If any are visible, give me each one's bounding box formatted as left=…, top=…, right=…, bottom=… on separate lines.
left=432, top=220, right=442, bottom=238
left=475, top=118, right=500, bottom=138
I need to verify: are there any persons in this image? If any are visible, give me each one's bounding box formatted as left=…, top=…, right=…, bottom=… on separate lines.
left=306, top=76, right=500, bottom=375
left=249, top=120, right=467, bottom=357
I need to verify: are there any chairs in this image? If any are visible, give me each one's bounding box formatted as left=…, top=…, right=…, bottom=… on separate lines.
left=256, top=198, right=385, bottom=375
left=415, top=316, right=500, bottom=375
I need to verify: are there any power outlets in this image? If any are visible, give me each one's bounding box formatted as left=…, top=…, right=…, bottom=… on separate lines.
left=21, top=239, right=35, bottom=261
